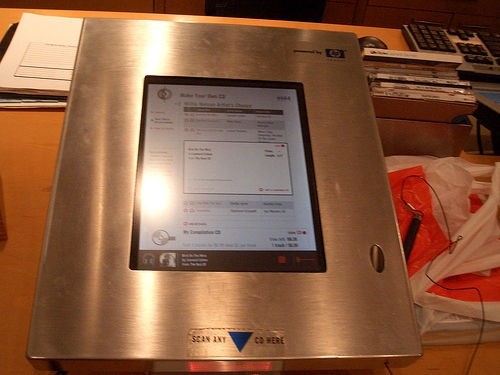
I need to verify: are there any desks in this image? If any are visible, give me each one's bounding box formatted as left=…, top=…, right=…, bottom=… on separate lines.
left=0, top=8, right=499, bottom=375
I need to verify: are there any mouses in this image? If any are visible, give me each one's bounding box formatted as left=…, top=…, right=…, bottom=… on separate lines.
left=358, top=36, right=388, bottom=50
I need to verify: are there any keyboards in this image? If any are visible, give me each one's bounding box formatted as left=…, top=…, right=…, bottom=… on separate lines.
left=401, top=21, right=500, bottom=81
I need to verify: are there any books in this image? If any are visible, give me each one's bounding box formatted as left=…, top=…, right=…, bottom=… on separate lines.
left=0, top=12, right=83, bottom=109
left=362, top=48, right=477, bottom=122
